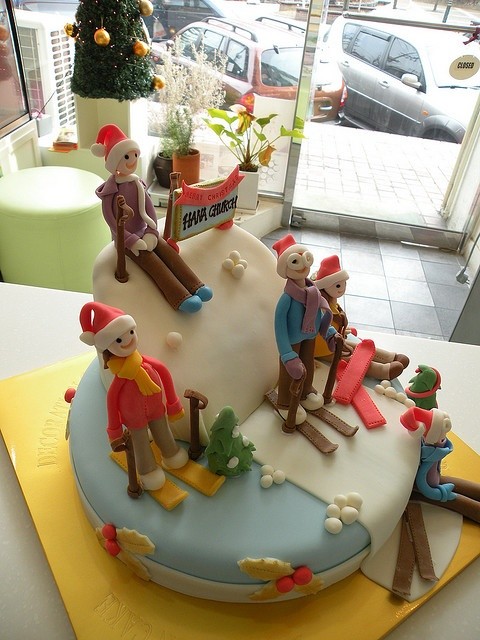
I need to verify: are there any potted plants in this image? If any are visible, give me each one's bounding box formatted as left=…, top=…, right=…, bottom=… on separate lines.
left=165, top=107, right=200, bottom=185
left=148, top=28, right=226, bottom=189
left=202, top=92, right=308, bottom=214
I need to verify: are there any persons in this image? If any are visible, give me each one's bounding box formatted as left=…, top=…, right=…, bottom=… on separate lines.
left=91, top=124, right=213, bottom=313
left=272, top=234, right=345, bottom=424
left=310, top=255, right=409, bottom=382
left=79, top=302, right=190, bottom=490
left=400, top=406, right=479, bottom=524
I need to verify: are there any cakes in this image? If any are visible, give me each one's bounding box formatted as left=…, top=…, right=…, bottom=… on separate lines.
left=64, top=122, right=480, bottom=602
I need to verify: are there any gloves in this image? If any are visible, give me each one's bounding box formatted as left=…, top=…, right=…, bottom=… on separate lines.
left=444, top=483, right=456, bottom=503
left=143, top=233, right=158, bottom=251
left=285, top=358, right=307, bottom=379
left=131, top=239, right=147, bottom=256
left=326, top=334, right=345, bottom=351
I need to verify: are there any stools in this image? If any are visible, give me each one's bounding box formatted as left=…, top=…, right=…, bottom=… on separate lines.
left=0, top=166, right=113, bottom=295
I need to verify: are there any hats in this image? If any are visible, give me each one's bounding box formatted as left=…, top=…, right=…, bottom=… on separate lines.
left=273, top=234, right=310, bottom=279
left=401, top=407, right=443, bottom=444
left=91, top=124, right=140, bottom=175
left=313, top=256, right=349, bottom=290
left=80, top=302, right=136, bottom=352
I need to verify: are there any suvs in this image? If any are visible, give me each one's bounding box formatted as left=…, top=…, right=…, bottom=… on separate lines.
left=328, top=9, right=480, bottom=143
left=160, top=15, right=348, bottom=126
